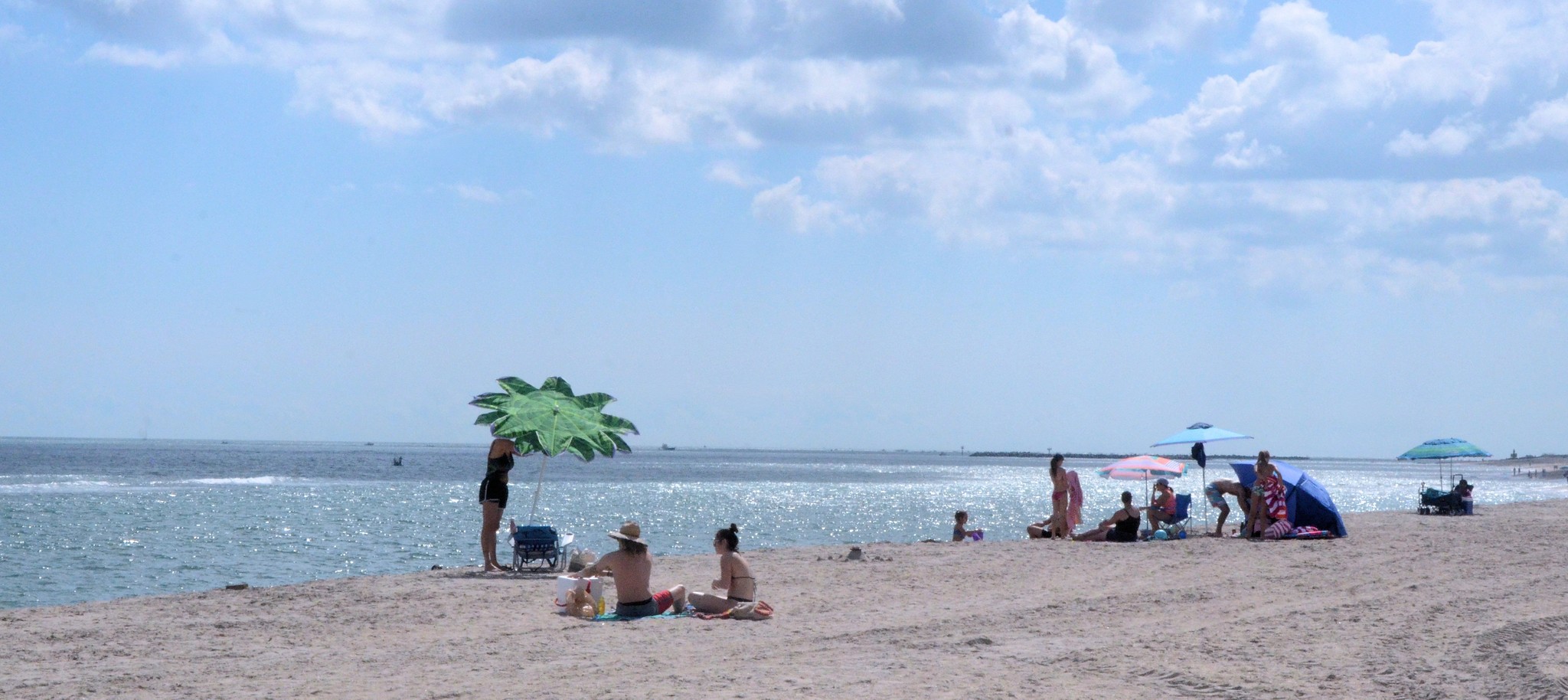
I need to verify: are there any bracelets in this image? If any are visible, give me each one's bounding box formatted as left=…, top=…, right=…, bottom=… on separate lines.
left=606, top=572, right=612, bottom=576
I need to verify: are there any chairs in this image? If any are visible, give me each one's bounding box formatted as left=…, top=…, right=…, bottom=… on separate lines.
left=1153, top=494, right=1193, bottom=541
left=507, top=521, right=574, bottom=572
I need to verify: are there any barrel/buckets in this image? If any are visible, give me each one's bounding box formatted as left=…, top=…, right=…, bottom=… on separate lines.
left=555, top=575, right=604, bottom=614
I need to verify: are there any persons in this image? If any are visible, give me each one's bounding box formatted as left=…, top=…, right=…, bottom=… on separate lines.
left=952, top=510, right=982, bottom=541
left=1554, top=464, right=1557, bottom=471
left=1027, top=515, right=1061, bottom=539
left=1513, top=467, right=1520, bottom=476
left=479, top=423, right=533, bottom=573
left=1069, top=491, right=1141, bottom=543
left=1147, top=478, right=1176, bottom=535
left=1454, top=480, right=1472, bottom=498
left=1245, top=450, right=1283, bottom=539
left=1049, top=454, right=1070, bottom=540
left=1205, top=477, right=1251, bottom=537
left=567, top=522, right=688, bottom=618
left=1528, top=468, right=1545, bottom=479
left=688, top=523, right=757, bottom=614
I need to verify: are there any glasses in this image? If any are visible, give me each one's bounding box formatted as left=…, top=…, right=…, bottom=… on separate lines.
left=713, top=539, right=723, bottom=545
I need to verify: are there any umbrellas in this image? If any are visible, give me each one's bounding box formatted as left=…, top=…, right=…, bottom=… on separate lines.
left=1396, top=438, right=1493, bottom=491
left=468, top=375, right=640, bottom=528
left=1228, top=460, right=1347, bottom=537
left=1150, top=421, right=1254, bottom=535
left=1097, top=453, right=1190, bottom=533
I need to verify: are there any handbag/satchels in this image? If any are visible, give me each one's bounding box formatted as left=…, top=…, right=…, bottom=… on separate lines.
left=568, top=546, right=597, bottom=573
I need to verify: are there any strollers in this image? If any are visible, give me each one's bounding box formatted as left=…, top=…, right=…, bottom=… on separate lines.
left=1416, top=474, right=1463, bottom=516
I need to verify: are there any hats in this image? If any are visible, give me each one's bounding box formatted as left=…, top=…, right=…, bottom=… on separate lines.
left=608, top=520, right=648, bottom=547
left=1157, top=478, right=1169, bottom=487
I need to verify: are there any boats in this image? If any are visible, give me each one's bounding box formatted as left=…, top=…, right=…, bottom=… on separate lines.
left=659, top=444, right=675, bottom=450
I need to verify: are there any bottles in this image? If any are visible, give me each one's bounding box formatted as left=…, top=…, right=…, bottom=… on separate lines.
left=598, top=596, right=605, bottom=615
left=1240, top=521, right=1244, bottom=534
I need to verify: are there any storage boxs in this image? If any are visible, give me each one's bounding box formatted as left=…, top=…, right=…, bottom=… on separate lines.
left=556, top=575, right=604, bottom=614
left=1461, top=496, right=1473, bottom=514
